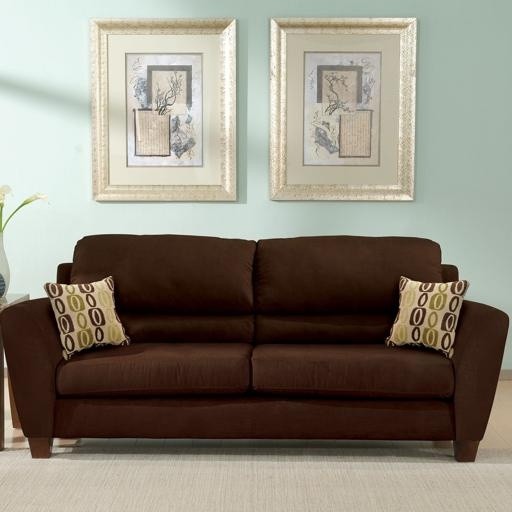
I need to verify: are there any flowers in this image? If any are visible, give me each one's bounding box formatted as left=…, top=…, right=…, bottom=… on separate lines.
left=0, top=184, right=48, bottom=234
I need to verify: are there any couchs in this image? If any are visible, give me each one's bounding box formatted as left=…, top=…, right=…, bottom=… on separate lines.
left=0, top=235, right=509, bottom=462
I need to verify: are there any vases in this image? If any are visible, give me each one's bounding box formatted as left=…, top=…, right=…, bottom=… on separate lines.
left=0, top=236, right=9, bottom=298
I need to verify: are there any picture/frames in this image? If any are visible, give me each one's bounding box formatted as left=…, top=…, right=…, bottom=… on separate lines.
left=268, top=17, right=416, bottom=201
left=91, top=18, right=236, bottom=202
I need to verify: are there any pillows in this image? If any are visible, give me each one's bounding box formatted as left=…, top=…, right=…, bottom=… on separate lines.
left=384, top=276, right=469, bottom=359
left=43, top=275, right=130, bottom=361
